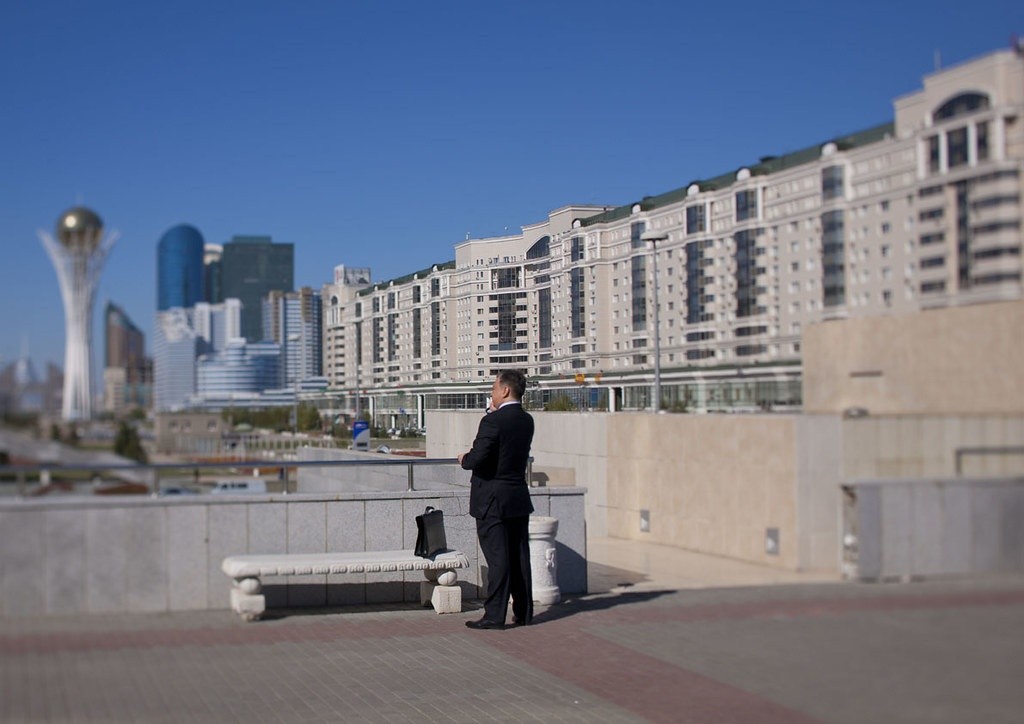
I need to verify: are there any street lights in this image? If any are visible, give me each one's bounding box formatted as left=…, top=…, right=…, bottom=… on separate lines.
left=352, top=317, right=363, bottom=421
left=639, top=228, right=671, bottom=413
left=288, top=333, right=301, bottom=440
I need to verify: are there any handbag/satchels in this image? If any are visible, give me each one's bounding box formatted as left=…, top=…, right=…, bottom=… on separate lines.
left=415, top=506, right=448, bottom=558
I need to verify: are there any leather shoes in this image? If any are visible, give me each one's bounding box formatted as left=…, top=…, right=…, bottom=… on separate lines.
left=466, top=617, right=503, bottom=630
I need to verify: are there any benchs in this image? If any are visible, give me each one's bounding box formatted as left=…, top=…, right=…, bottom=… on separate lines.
left=221, top=549, right=472, bottom=622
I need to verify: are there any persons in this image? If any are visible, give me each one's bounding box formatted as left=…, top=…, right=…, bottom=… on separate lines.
left=458, top=370, right=534, bottom=631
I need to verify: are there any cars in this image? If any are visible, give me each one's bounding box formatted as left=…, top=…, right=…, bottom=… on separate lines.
left=159, top=486, right=202, bottom=496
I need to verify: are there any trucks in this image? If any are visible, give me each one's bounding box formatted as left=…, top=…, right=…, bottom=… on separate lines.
left=210, top=478, right=269, bottom=497
